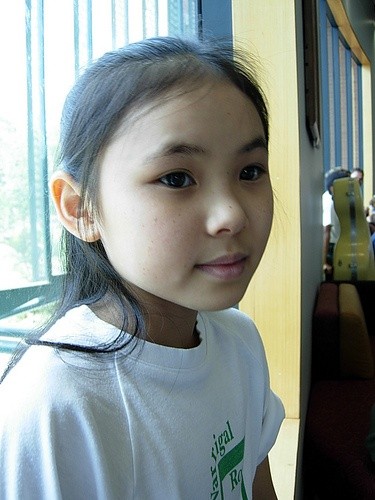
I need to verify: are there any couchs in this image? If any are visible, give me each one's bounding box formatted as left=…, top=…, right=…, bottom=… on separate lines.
left=308, top=281, right=375, bottom=500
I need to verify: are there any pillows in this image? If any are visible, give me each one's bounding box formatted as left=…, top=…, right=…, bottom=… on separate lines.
left=338, top=284, right=373, bottom=378
left=312, top=282, right=339, bottom=368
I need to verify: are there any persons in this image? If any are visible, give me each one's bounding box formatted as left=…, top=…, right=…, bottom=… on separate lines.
left=0, top=36, right=285, bottom=500
left=321, top=167, right=375, bottom=282
